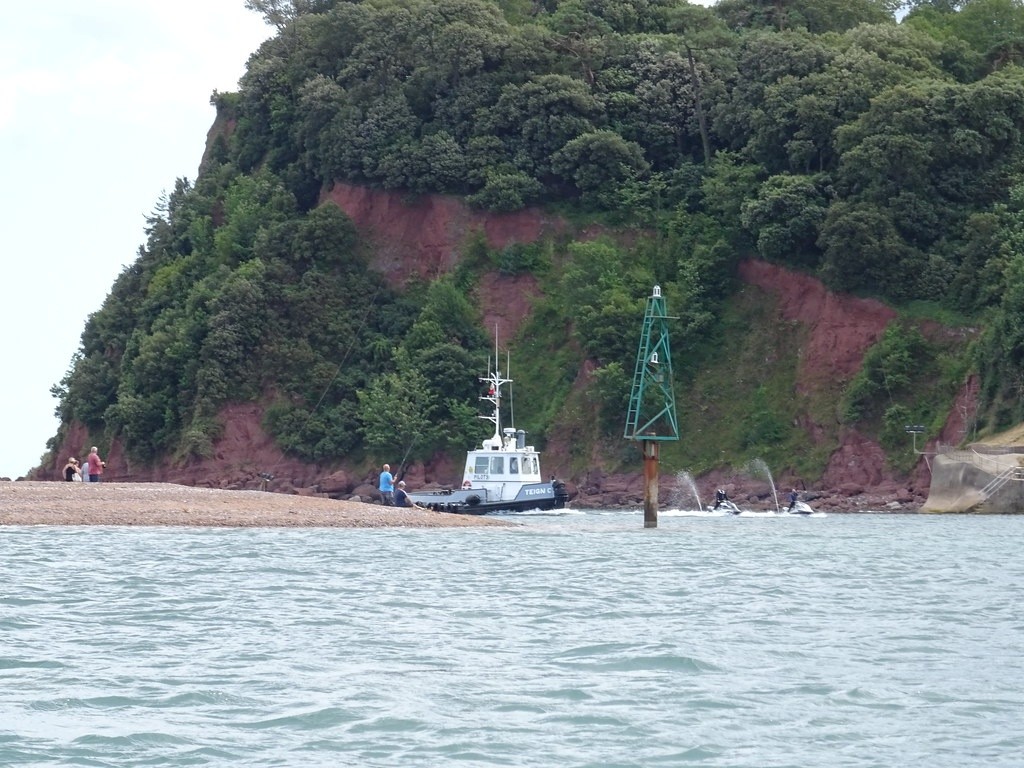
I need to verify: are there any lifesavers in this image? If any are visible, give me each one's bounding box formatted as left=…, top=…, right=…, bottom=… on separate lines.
left=438, top=504, right=446, bottom=512
left=552, top=480, right=569, bottom=502
left=447, top=504, right=454, bottom=513
left=416, top=501, right=423, bottom=506
left=428, top=503, right=436, bottom=511
left=463, top=481, right=471, bottom=490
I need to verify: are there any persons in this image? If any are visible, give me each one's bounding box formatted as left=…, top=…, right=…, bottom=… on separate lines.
left=87, top=446, right=105, bottom=482
left=788, top=489, right=798, bottom=512
left=375, top=464, right=397, bottom=505
left=80, top=459, right=90, bottom=482
left=62, top=457, right=81, bottom=481
left=714, top=487, right=728, bottom=510
left=395, top=481, right=413, bottom=507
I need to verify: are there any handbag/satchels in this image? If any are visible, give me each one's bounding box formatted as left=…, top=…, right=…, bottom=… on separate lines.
left=72, top=473, right=82, bottom=482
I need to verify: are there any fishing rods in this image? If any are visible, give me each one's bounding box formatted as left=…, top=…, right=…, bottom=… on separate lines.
left=393, top=407, right=433, bottom=477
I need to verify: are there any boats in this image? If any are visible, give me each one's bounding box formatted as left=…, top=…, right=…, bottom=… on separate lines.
left=399, top=323, right=569, bottom=517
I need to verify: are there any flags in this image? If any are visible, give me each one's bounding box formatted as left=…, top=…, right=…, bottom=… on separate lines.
left=489, top=383, right=495, bottom=395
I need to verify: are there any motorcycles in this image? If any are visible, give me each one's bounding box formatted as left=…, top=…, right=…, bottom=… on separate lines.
left=782, top=501, right=814, bottom=516
left=707, top=500, right=741, bottom=515
